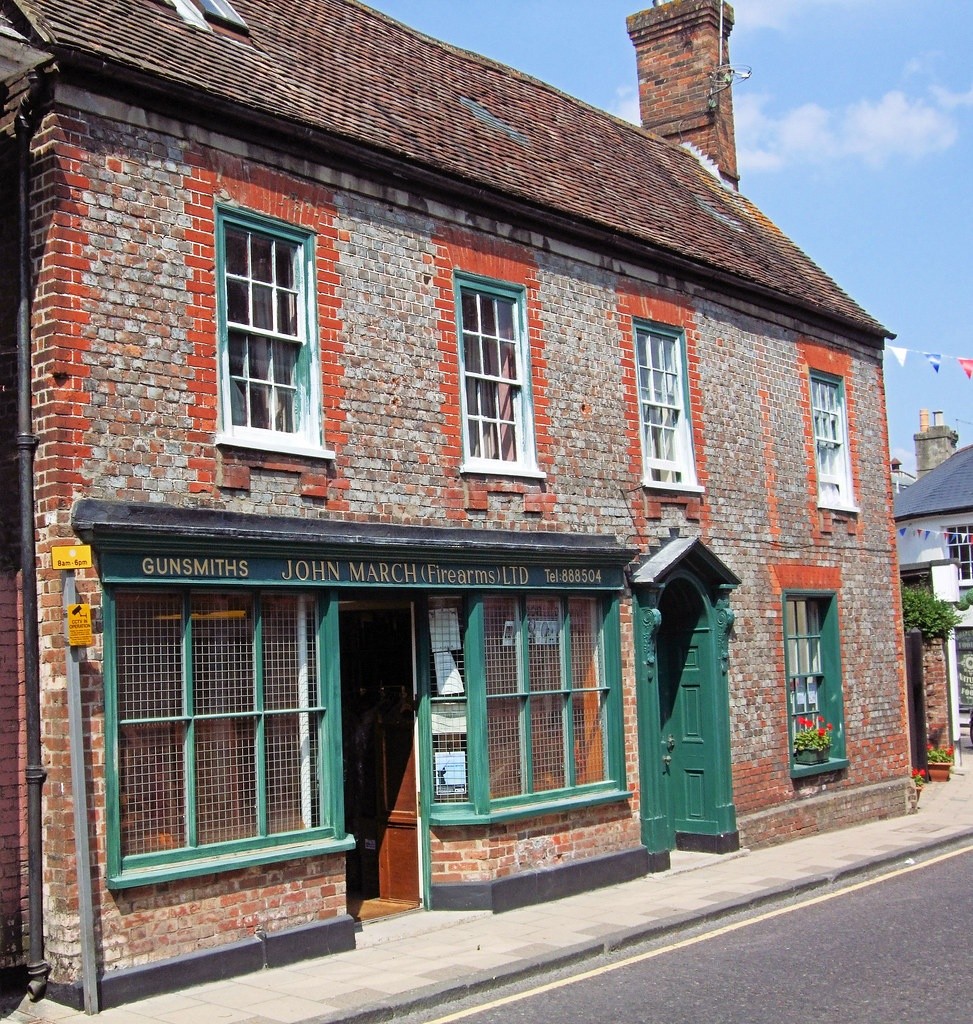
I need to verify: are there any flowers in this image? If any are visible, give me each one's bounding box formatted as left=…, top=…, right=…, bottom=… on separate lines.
left=927, top=743, right=955, bottom=763
left=793, top=716, right=833, bottom=752
left=912, top=768, right=926, bottom=787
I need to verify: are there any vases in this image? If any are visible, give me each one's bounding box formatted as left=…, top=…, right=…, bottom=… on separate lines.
left=928, top=761, right=951, bottom=782
left=796, top=745, right=831, bottom=765
left=916, top=787, right=922, bottom=801
